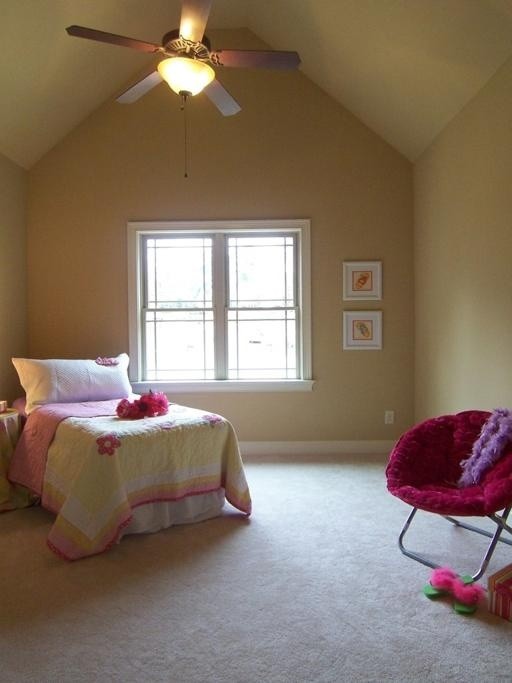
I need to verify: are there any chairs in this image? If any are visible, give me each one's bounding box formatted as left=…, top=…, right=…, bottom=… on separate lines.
left=383, top=407, right=512, bottom=586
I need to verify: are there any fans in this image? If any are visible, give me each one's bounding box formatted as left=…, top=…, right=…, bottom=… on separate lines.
left=63, top=0, right=304, bottom=121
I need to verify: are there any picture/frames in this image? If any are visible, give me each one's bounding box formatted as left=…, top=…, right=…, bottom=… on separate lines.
left=340, top=258, right=386, bottom=352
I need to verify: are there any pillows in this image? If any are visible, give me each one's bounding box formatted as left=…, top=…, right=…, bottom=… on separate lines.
left=9, top=352, right=140, bottom=414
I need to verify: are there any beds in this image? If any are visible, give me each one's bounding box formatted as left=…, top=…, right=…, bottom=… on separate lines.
left=13, top=350, right=230, bottom=540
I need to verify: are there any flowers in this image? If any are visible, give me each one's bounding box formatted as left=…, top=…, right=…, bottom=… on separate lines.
left=114, top=389, right=176, bottom=420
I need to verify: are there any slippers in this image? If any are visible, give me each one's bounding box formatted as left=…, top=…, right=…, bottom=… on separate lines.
left=455, top=582, right=484, bottom=613
left=423, top=568, right=472, bottom=598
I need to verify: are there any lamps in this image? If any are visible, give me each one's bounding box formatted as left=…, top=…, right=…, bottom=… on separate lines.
left=154, top=49, right=215, bottom=101
